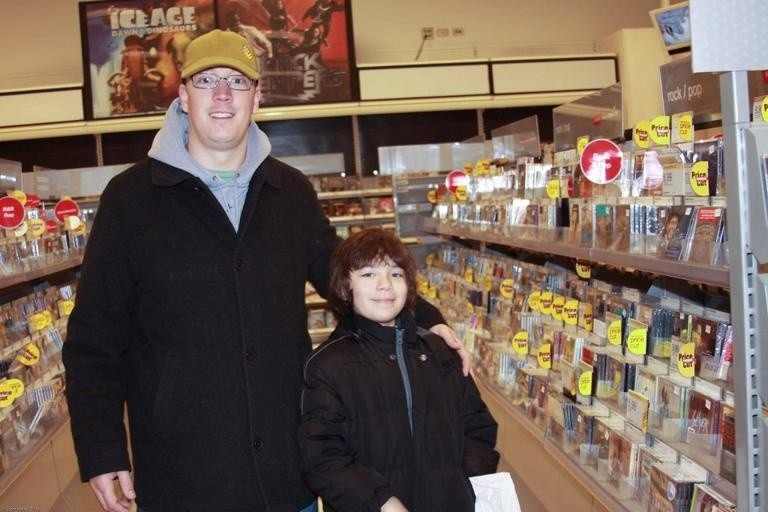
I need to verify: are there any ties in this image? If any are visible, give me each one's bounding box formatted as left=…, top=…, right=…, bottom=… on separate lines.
left=647, top=1, right=690, bottom=52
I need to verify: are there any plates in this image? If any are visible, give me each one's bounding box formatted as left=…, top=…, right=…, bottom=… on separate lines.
left=1, top=161, right=125, bottom=509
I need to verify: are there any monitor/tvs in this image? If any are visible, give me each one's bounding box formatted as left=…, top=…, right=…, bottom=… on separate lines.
left=0, top=226, right=90, bottom=477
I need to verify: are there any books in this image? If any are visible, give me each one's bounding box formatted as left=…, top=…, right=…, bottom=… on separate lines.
left=0, top=226, right=90, bottom=477
left=428, top=139, right=737, bottom=512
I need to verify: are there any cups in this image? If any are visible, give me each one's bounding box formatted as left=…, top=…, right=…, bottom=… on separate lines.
left=192, top=72, right=253, bottom=91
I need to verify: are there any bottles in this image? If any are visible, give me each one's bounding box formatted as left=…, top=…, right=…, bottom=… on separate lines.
left=180, top=28, right=261, bottom=82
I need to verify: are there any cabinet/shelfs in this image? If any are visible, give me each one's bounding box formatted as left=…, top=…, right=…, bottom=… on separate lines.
left=1, top=161, right=125, bottom=509
left=414, top=146, right=768, bottom=512
left=301, top=170, right=403, bottom=344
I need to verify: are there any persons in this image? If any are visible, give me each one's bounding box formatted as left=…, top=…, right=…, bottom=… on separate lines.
left=63, top=29, right=477, bottom=512
left=298, top=228, right=499, bottom=512
left=270, top=0, right=345, bottom=63
left=226, top=11, right=273, bottom=73
left=660, top=213, right=682, bottom=252
left=664, top=24, right=680, bottom=40
